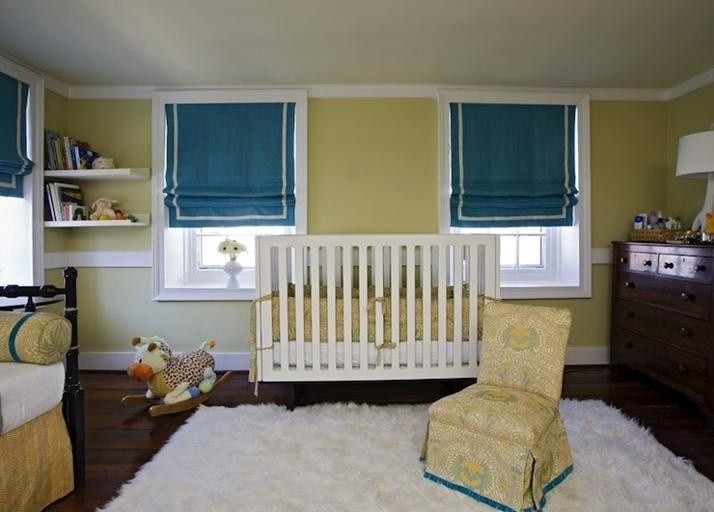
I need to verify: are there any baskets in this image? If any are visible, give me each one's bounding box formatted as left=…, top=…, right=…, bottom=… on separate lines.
left=631, top=229, right=674, bottom=242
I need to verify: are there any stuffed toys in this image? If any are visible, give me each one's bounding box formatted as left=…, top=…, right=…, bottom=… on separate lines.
left=90, top=198, right=119, bottom=220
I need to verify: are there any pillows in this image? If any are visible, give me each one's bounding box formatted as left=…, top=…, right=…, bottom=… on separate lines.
left=0, top=311, right=73, bottom=366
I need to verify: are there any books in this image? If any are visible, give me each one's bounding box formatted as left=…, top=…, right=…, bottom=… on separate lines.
left=44, top=128, right=97, bottom=220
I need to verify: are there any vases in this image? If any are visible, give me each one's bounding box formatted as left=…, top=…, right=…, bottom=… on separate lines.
left=223, top=259, right=243, bottom=288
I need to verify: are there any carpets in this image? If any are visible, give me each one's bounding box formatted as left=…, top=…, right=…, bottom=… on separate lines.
left=94, top=403, right=714, bottom=512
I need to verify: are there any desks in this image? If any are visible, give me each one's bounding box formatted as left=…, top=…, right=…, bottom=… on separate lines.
left=0, top=295, right=66, bottom=311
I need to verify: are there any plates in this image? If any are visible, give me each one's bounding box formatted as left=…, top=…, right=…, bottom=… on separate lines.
left=667, top=239, right=685, bottom=244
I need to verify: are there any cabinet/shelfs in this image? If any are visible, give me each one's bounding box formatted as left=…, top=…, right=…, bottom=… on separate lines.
left=43, top=167, right=151, bottom=229
left=609, top=239, right=713, bottom=428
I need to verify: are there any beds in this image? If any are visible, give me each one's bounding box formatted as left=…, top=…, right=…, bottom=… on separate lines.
left=0, top=266, right=84, bottom=511
left=253, top=232, right=502, bottom=411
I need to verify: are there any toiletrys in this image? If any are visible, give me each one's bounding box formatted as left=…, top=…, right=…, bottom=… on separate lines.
left=649, top=211, right=656, bottom=223
left=633, top=208, right=682, bottom=231
left=634, top=216, right=643, bottom=230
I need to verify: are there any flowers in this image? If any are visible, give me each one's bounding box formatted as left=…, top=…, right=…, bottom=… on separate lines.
left=218, top=239, right=247, bottom=261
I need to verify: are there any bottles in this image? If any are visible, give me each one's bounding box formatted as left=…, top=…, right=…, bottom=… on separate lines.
left=634, top=209, right=682, bottom=230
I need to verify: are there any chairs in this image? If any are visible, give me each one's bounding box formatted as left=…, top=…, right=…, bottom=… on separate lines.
left=422, top=301, right=573, bottom=511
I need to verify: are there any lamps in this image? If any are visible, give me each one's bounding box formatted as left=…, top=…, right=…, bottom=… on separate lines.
left=675, top=130, right=713, bottom=235
